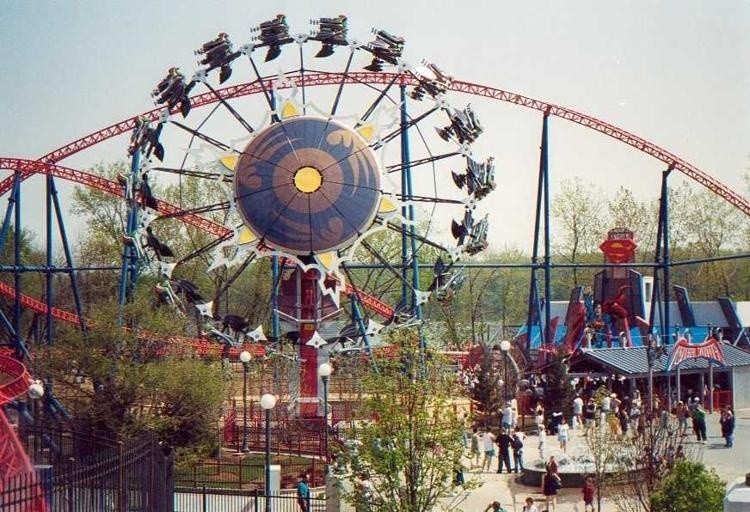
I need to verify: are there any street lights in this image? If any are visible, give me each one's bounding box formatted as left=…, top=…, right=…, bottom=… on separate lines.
left=261, top=393, right=277, bottom=511
left=318, top=363, right=334, bottom=456
left=28, top=383, right=44, bottom=463
left=240, top=350, right=253, bottom=451
left=499, top=339, right=511, bottom=401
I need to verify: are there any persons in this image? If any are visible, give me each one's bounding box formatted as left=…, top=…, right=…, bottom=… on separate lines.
left=296, top=474, right=313, bottom=512
left=118, top=13, right=498, bottom=367
left=581, top=286, right=612, bottom=349
left=326, top=361, right=736, bottom=512
